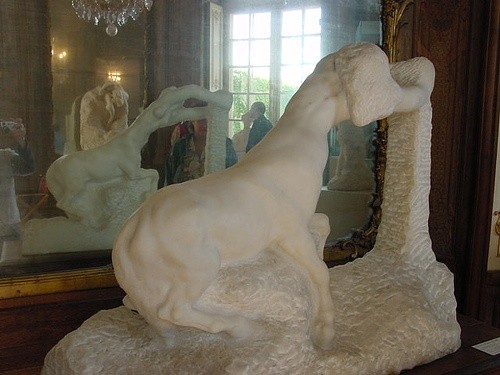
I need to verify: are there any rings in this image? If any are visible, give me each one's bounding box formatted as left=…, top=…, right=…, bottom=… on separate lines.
left=19, top=128, right=24, bottom=132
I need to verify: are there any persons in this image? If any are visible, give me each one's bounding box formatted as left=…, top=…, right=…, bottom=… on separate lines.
left=232, top=112, right=254, bottom=160
left=245, top=102, right=274, bottom=154
left=163, top=99, right=238, bottom=187
left=0, top=117, right=36, bottom=225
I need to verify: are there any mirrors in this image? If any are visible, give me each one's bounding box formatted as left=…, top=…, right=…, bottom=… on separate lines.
left=0, top=0, right=417, bottom=304
